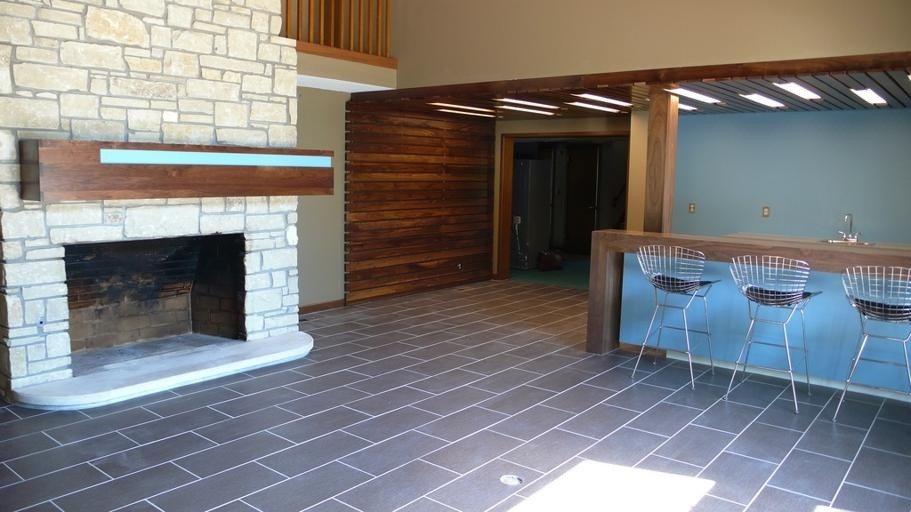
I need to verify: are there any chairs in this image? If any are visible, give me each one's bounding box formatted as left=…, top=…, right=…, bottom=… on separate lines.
left=631, top=244, right=722, bottom=392
left=427, top=81, right=888, bottom=119
left=722, top=255, right=823, bottom=415
left=832, top=264, right=910, bottom=420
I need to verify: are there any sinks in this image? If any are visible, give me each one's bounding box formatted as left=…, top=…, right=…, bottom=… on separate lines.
left=821, top=241, right=875, bottom=248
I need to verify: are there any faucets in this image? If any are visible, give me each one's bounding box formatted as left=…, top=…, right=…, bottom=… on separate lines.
left=844, top=213, right=854, bottom=240
